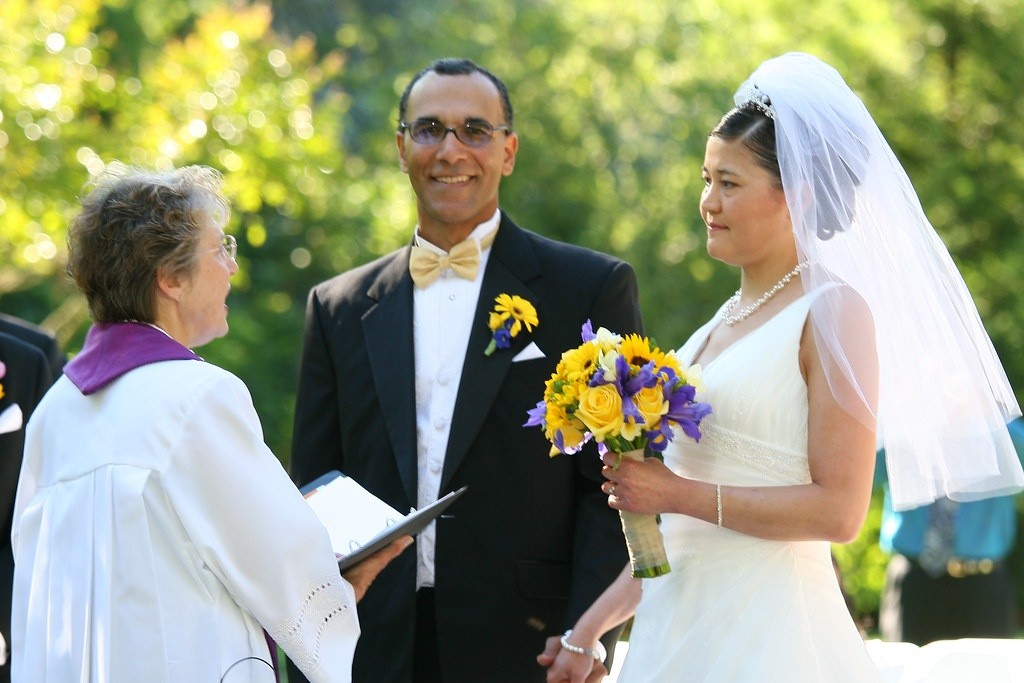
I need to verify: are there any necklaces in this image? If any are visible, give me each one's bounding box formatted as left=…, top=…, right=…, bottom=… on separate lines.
left=724, top=254, right=816, bottom=330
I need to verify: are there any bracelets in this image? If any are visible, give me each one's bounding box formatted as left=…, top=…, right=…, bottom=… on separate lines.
left=716, top=483, right=723, bottom=528
left=560, top=629, right=601, bottom=662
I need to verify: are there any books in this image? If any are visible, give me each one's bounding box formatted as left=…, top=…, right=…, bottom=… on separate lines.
left=299, top=468, right=469, bottom=568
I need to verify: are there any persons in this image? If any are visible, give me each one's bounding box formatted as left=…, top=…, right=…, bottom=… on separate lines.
left=548, top=52, right=1023, bottom=681
left=287, top=58, right=650, bottom=682
left=1, top=310, right=71, bottom=683
left=11, top=162, right=419, bottom=683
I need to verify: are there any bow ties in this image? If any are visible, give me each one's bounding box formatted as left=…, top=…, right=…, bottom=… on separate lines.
left=409, top=222, right=500, bottom=289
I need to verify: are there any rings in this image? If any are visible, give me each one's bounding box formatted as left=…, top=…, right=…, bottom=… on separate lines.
left=609, top=486, right=617, bottom=497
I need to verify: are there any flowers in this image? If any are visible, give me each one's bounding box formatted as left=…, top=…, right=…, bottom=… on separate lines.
left=0, top=360, right=7, bottom=400
left=485, top=292, right=539, bottom=356
left=522, top=319, right=713, bottom=579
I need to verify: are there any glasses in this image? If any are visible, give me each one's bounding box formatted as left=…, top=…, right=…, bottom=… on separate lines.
left=154, top=235, right=237, bottom=278
left=400, top=120, right=510, bottom=145
